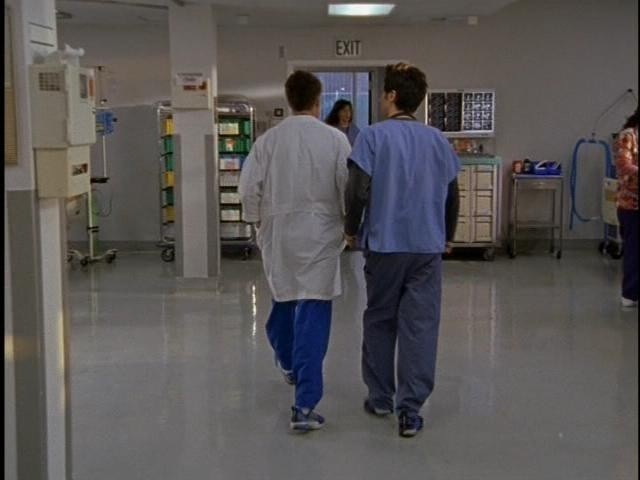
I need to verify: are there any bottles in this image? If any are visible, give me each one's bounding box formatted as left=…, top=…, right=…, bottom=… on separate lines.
left=523, top=158, right=532, bottom=174
left=512, top=160, right=523, bottom=174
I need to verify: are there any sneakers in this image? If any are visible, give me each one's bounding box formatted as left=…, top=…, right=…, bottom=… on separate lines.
left=290, top=405, right=325, bottom=430
left=399, top=412, right=423, bottom=436
left=622, top=296, right=638, bottom=306
left=364, top=398, right=392, bottom=416
left=275, top=354, right=296, bottom=386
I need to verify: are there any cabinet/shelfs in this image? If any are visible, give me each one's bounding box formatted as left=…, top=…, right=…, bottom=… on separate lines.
left=507, top=171, right=565, bottom=259
left=156, top=101, right=254, bottom=262
left=452, top=154, right=502, bottom=261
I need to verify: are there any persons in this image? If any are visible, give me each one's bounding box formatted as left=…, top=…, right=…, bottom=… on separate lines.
left=238, top=70, right=354, bottom=430
left=324, top=98, right=363, bottom=251
left=611, top=107, right=639, bottom=308
left=345, top=63, right=460, bottom=438
